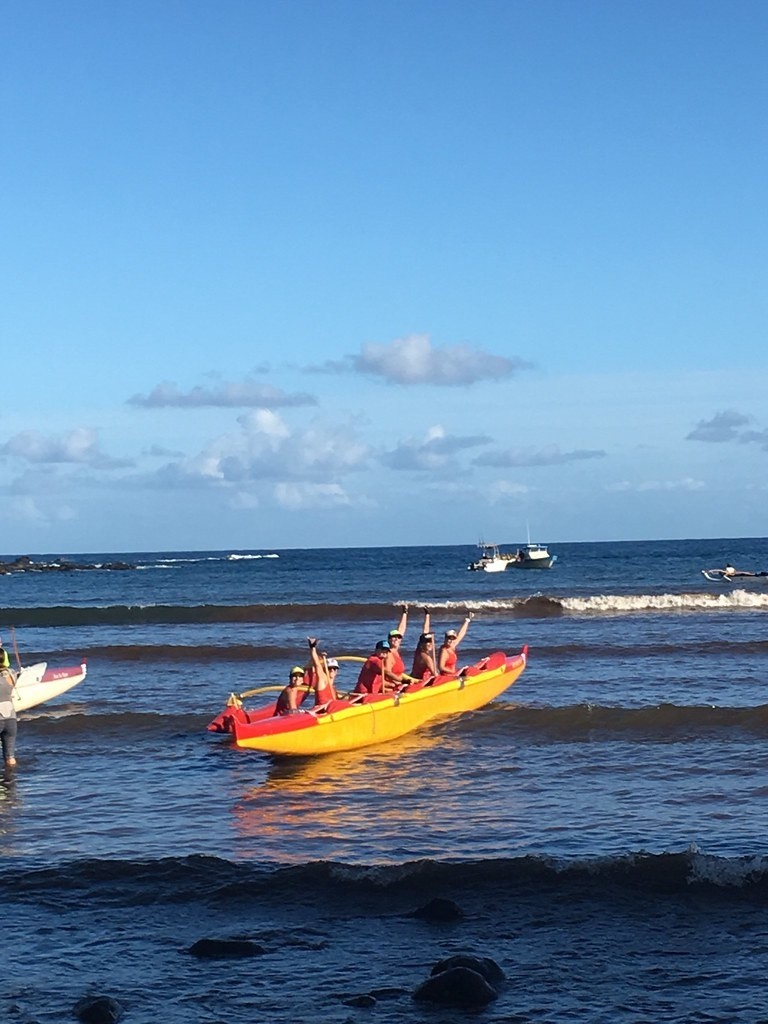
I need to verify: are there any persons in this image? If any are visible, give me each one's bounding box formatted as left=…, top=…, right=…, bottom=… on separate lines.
left=308, top=637, right=341, bottom=713
left=0, top=645, right=17, bottom=766
left=725, top=563, right=736, bottom=577
left=438, top=611, right=475, bottom=676
left=274, top=666, right=305, bottom=716
left=410, top=606, right=437, bottom=682
left=353, top=640, right=403, bottom=695
left=383, top=602, right=408, bottom=692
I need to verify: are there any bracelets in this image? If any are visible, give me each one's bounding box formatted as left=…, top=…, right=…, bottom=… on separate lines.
left=464, top=618, right=471, bottom=622
left=403, top=609, right=408, bottom=614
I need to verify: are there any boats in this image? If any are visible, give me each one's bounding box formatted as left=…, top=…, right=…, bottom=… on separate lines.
left=207, top=645, right=527, bottom=757
left=512, top=521, right=557, bottom=568
left=702, top=568, right=768, bottom=587
left=468, top=540, right=516, bottom=572
left=9, top=627, right=87, bottom=714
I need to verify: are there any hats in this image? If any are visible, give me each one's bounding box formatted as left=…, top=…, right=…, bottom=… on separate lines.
left=290, top=667, right=304, bottom=674
left=420, top=633, right=432, bottom=642
left=388, top=630, right=403, bottom=639
left=376, top=640, right=391, bottom=652
left=327, top=659, right=340, bottom=668
left=445, top=630, right=457, bottom=639
